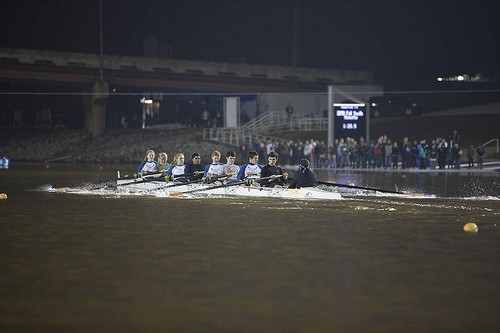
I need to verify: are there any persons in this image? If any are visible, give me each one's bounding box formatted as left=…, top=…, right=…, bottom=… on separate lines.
left=467, top=144, right=475, bottom=169
left=237, top=150, right=265, bottom=181
left=221, top=151, right=240, bottom=183
left=329, top=135, right=462, bottom=170
left=204, top=151, right=225, bottom=177
left=184, top=153, right=206, bottom=179
left=241, top=136, right=328, bottom=170
left=138, top=150, right=158, bottom=174
left=477, top=144, right=485, bottom=169
left=288, top=159, right=318, bottom=189
left=152, top=152, right=171, bottom=172
left=167, top=153, right=186, bottom=177
left=260, top=152, right=288, bottom=188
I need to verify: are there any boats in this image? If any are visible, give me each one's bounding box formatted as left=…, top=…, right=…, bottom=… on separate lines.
left=114, top=170, right=348, bottom=203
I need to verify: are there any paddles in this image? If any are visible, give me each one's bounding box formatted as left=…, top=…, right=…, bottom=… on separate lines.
left=58, top=172, right=420, bottom=197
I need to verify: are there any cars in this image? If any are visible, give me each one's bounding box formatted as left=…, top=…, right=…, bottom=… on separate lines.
left=369, top=94, right=426, bottom=117
